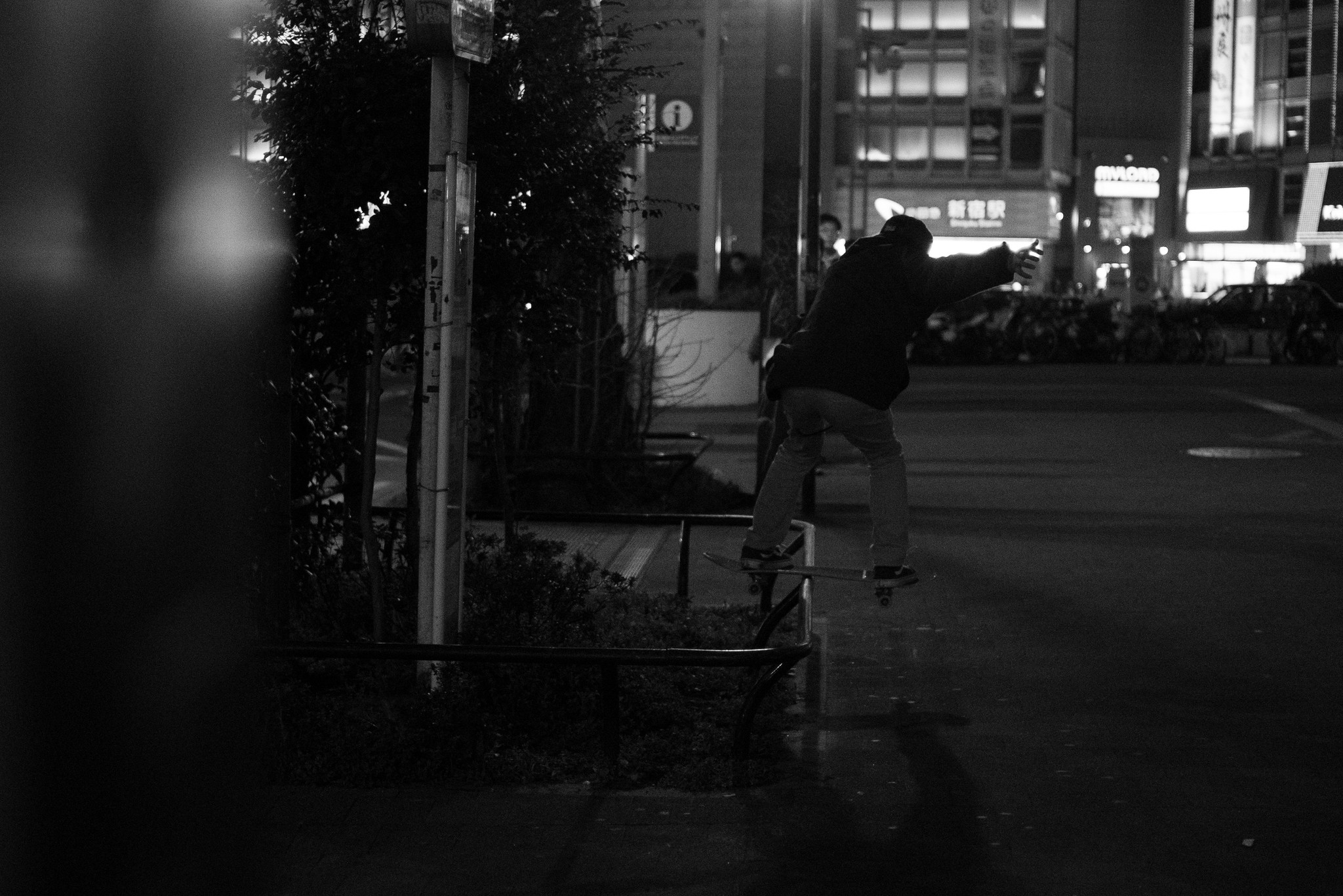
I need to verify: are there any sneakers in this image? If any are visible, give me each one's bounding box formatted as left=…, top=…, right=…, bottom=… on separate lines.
left=875, top=566, right=920, bottom=589
left=736, top=546, right=794, bottom=569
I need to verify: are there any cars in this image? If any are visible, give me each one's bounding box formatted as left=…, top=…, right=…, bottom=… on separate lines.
left=928, top=283, right=1343, bottom=365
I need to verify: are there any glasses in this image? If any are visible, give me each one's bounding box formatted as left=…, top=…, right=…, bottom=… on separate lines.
left=819, top=228, right=840, bottom=235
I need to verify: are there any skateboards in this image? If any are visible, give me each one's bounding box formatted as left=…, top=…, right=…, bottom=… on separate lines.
left=701, top=551, right=922, bottom=608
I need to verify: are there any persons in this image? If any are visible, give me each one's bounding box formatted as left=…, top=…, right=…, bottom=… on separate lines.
left=818, top=212, right=841, bottom=268
left=722, top=251, right=755, bottom=288
left=672, top=252, right=700, bottom=290
left=738, top=215, right=1045, bottom=577
